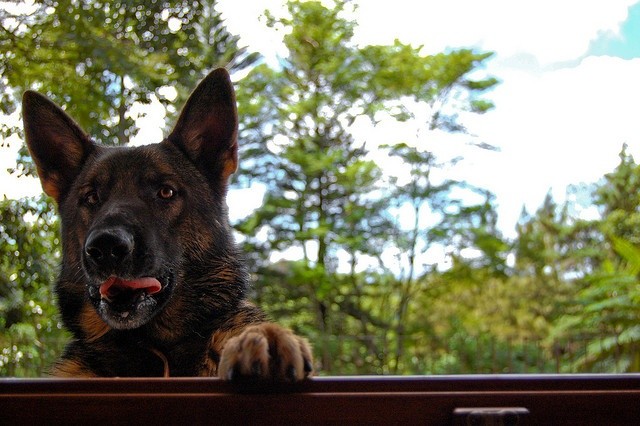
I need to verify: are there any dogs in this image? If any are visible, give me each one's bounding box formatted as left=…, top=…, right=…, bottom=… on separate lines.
left=22, top=67, right=315, bottom=381
